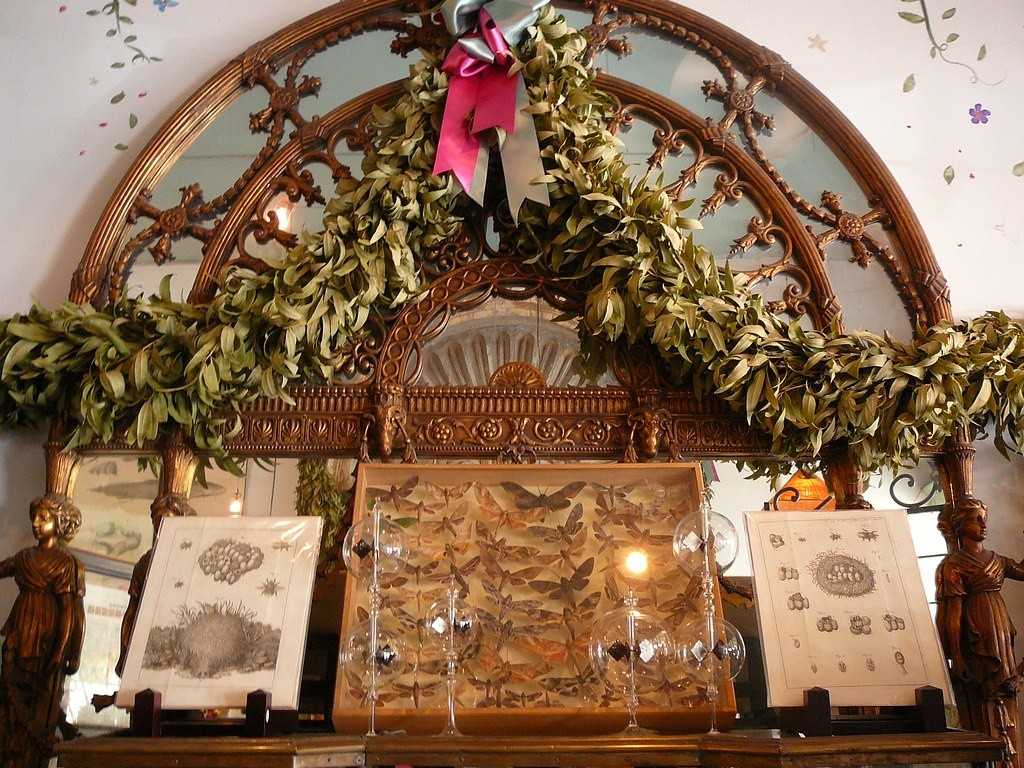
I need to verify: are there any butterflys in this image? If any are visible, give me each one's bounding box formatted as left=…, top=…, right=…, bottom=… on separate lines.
left=344, top=476, right=708, bottom=710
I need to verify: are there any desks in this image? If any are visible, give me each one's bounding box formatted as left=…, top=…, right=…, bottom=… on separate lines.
left=42, top=731, right=1008, bottom=768
